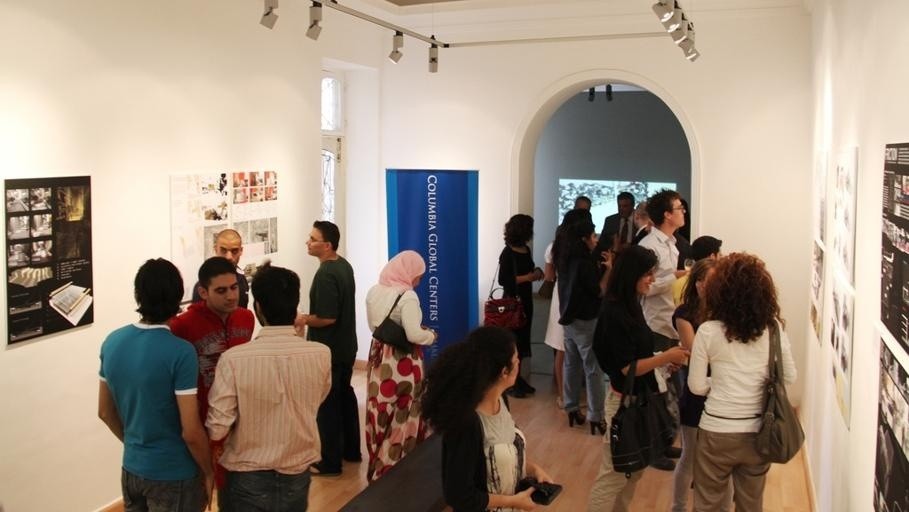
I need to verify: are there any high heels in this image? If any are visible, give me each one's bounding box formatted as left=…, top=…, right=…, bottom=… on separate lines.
left=567, top=409, right=586, bottom=428
left=589, top=419, right=608, bottom=437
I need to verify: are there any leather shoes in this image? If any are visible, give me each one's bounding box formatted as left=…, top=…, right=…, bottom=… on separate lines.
left=506, top=377, right=536, bottom=399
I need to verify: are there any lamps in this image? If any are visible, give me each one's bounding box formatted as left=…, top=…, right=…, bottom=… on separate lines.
left=306, top=2, right=322, bottom=39
left=651, top=0, right=699, bottom=63
left=389, top=31, right=403, bottom=64
left=259, top=0, right=279, bottom=30
left=429, top=35, right=438, bottom=72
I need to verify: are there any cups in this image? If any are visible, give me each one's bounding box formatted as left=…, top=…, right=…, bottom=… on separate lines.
left=684, top=258, right=696, bottom=272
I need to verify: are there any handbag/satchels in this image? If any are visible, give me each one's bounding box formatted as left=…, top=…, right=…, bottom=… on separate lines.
left=756, top=380, right=805, bottom=465
left=484, top=296, right=523, bottom=330
left=610, top=394, right=677, bottom=474
left=372, top=318, right=415, bottom=355
left=537, top=275, right=557, bottom=301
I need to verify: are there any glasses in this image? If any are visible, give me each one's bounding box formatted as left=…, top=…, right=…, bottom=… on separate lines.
left=671, top=205, right=684, bottom=212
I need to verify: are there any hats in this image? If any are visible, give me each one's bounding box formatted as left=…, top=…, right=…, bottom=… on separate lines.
left=690, top=236, right=722, bottom=262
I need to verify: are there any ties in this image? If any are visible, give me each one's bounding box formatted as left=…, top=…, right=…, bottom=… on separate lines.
left=618, top=217, right=629, bottom=247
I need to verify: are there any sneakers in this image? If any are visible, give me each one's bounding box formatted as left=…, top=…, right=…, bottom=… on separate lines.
left=309, top=460, right=342, bottom=476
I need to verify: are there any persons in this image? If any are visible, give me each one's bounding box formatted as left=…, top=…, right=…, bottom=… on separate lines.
left=167, top=257, right=255, bottom=512
left=193, top=229, right=249, bottom=309
left=365, top=250, right=438, bottom=486
left=421, top=326, right=554, bottom=512
left=687, top=252, right=796, bottom=512
left=671, top=258, right=718, bottom=512
left=294, top=221, right=362, bottom=476
left=499, top=213, right=545, bottom=398
left=98, top=258, right=215, bottom=512
left=545, top=190, right=721, bottom=472
left=205, top=267, right=332, bottom=512
left=587, top=245, right=691, bottom=512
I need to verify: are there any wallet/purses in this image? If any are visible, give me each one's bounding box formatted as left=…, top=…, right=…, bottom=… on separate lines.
left=517, top=477, right=562, bottom=504
